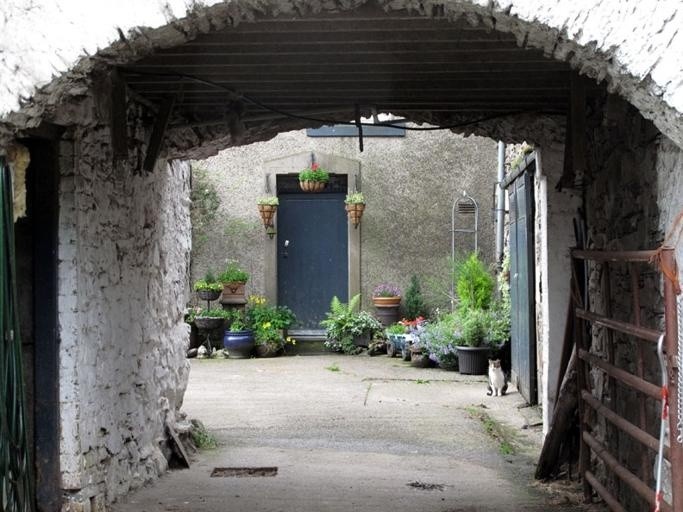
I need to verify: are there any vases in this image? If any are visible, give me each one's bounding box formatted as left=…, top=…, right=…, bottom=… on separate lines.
left=273, top=328, right=287, bottom=339
left=371, top=297, right=400, bottom=326
left=299, top=181, right=324, bottom=192
left=257, top=340, right=281, bottom=357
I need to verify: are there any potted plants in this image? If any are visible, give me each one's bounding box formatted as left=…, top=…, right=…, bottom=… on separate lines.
left=184, top=264, right=255, bottom=360
left=343, top=190, right=367, bottom=229
left=452, top=248, right=497, bottom=375
left=257, top=194, right=279, bottom=231
left=319, top=293, right=383, bottom=355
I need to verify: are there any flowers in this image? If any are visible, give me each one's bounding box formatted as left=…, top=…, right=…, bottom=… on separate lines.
left=298, top=163, right=328, bottom=184
left=245, top=293, right=297, bottom=349
left=405, top=311, right=463, bottom=368
left=371, top=282, right=401, bottom=298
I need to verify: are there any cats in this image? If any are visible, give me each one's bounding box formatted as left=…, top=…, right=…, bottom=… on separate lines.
left=486, top=358, right=508, bottom=397
